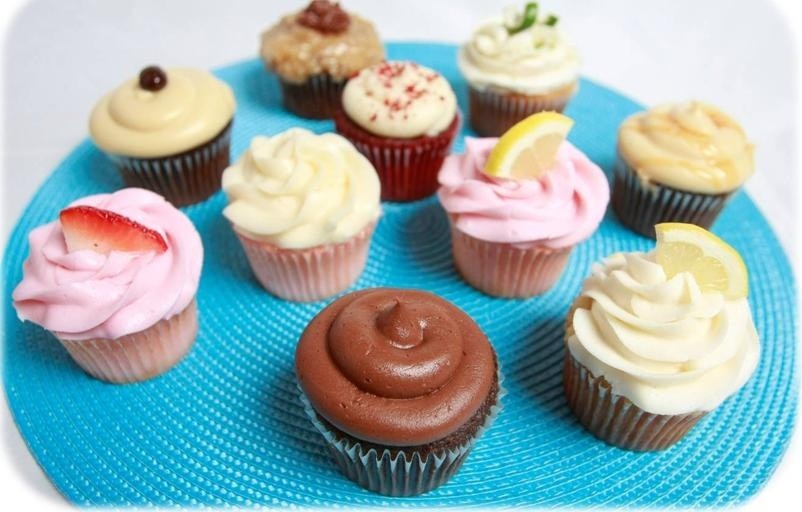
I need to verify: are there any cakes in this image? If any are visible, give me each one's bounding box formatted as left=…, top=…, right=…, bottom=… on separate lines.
left=259, top=2, right=382, bottom=121
left=12, top=186, right=204, bottom=385
left=456, top=4, right=577, bottom=138
left=562, top=223, right=761, bottom=454
left=335, top=60, right=462, bottom=203
left=219, top=128, right=383, bottom=304
left=91, top=65, right=235, bottom=209
left=610, top=97, right=753, bottom=240
left=294, top=288, right=505, bottom=499
left=435, top=112, right=610, bottom=299
